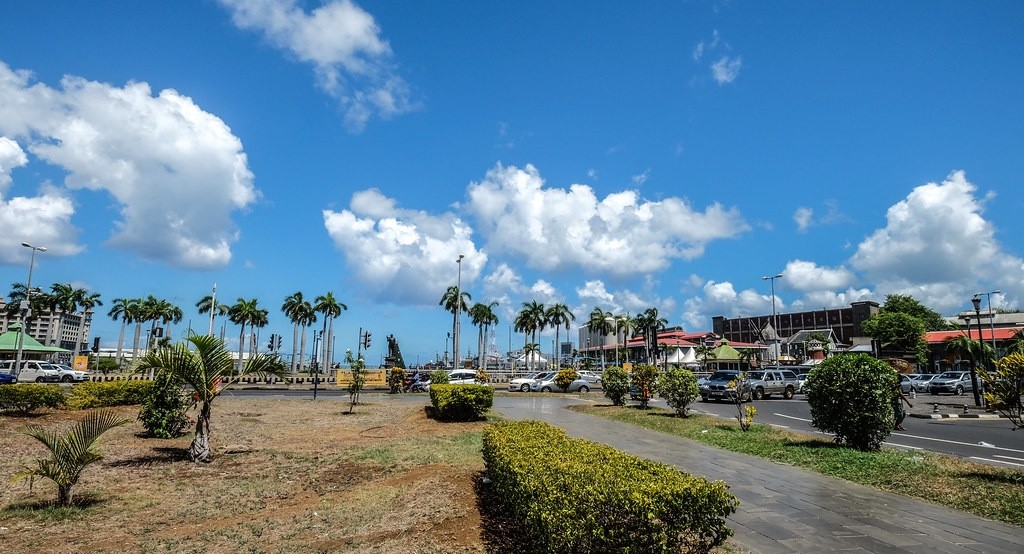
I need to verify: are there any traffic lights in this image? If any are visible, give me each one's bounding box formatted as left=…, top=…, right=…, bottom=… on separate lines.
left=91, top=337, right=99, bottom=353
left=365, top=330, right=372, bottom=350
left=152, top=327, right=157, bottom=335
left=268, top=334, right=282, bottom=351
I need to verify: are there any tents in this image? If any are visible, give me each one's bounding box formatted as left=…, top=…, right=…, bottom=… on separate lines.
left=658, top=347, right=705, bottom=371
left=518, top=350, right=547, bottom=369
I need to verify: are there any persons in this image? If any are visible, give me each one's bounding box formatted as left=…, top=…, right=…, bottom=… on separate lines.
left=405, top=370, right=419, bottom=392
left=892, top=375, right=913, bottom=431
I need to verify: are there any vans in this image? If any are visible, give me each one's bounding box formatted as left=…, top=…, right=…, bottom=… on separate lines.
left=744, top=369, right=799, bottom=400
left=929, top=371, right=981, bottom=396
left=0, top=360, right=60, bottom=383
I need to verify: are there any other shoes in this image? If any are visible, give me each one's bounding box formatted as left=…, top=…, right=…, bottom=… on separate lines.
left=894, top=427, right=904, bottom=431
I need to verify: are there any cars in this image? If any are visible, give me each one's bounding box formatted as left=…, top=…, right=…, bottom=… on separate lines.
left=530, top=370, right=602, bottom=393
left=0, top=373, right=18, bottom=385
left=508, top=371, right=559, bottom=392
left=765, top=366, right=814, bottom=394
left=900, top=374, right=947, bottom=394
left=404, top=369, right=489, bottom=393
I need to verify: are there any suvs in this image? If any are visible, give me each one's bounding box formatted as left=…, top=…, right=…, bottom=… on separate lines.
left=41, top=364, right=90, bottom=383
left=697, top=370, right=753, bottom=402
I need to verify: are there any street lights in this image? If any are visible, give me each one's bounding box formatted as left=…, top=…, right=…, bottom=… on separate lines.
left=605, top=317, right=627, bottom=366
left=15, top=242, right=47, bottom=376
left=964, top=290, right=1000, bottom=406
left=762, top=274, right=783, bottom=368
left=456, top=254, right=465, bottom=368
